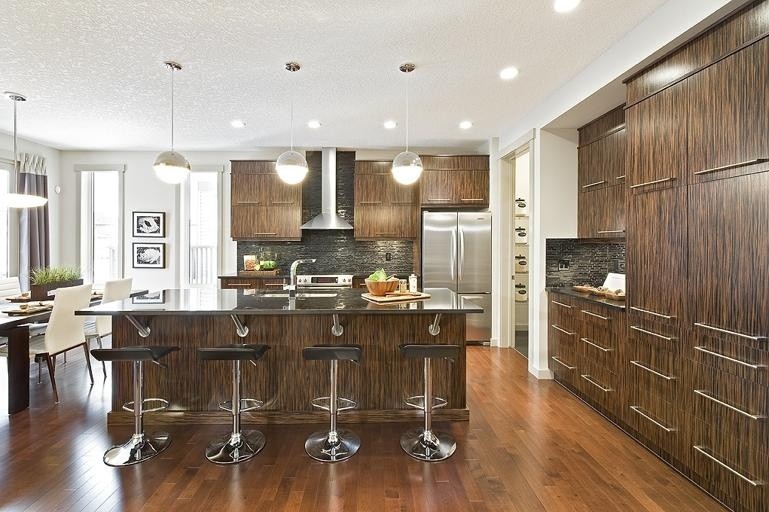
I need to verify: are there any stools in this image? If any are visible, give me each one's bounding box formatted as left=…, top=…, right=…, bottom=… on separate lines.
left=398, top=343, right=460, bottom=463
left=197, top=343, right=269, bottom=464
left=301, top=344, right=362, bottom=463
left=90, top=344, right=182, bottom=467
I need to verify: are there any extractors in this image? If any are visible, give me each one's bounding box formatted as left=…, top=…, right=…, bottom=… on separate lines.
left=298, top=148, right=354, bottom=231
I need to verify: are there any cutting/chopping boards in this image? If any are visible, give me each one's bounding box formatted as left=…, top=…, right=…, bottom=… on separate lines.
left=238, top=270, right=280, bottom=276
left=360, top=291, right=431, bottom=303
left=359, top=296, right=431, bottom=306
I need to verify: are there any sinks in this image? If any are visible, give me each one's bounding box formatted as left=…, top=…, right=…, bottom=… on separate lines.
left=260, top=293, right=339, bottom=298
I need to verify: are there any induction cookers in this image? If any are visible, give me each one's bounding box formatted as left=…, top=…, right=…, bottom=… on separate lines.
left=296, top=272, right=353, bottom=286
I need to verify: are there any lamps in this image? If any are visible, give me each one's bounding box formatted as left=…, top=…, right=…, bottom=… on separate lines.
left=391, top=62, right=424, bottom=186
left=152, top=60, right=191, bottom=185
left=0, top=91, right=48, bottom=209
left=274, top=61, right=310, bottom=185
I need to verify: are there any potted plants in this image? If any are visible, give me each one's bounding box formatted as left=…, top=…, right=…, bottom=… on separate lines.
left=29, top=263, right=83, bottom=301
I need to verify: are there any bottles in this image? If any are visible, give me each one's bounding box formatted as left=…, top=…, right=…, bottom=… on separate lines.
left=409, top=273, right=418, bottom=293
left=514, top=254, right=527, bottom=273
left=515, top=283, right=528, bottom=302
left=514, top=226, right=527, bottom=244
left=515, top=197, right=527, bottom=216
left=398, top=280, right=408, bottom=293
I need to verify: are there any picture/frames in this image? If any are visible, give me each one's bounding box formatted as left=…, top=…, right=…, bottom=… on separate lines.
left=131, top=291, right=166, bottom=305
left=132, top=211, right=165, bottom=238
left=132, top=242, right=165, bottom=269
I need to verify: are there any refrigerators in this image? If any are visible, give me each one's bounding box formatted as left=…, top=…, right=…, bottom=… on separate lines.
left=419, top=209, right=492, bottom=343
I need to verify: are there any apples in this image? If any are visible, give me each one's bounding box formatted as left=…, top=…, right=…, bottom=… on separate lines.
left=369, top=271, right=386, bottom=281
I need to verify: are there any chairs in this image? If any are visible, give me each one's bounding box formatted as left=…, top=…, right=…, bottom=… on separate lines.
left=0, top=277, right=132, bottom=416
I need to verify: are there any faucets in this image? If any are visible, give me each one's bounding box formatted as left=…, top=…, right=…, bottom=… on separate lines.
left=289, top=259, right=316, bottom=291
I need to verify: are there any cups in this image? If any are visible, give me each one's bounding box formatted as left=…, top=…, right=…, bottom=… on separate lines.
left=243, top=254, right=277, bottom=271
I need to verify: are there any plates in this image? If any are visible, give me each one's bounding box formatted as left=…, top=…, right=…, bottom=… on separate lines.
left=574, top=285, right=625, bottom=300
left=2, top=304, right=52, bottom=316
left=5, top=296, right=31, bottom=302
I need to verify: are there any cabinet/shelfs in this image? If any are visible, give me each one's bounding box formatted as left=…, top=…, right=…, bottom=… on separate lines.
left=353, top=162, right=421, bottom=242
left=419, top=157, right=489, bottom=208
left=231, top=162, right=303, bottom=243
left=548, top=289, right=625, bottom=426
left=575, top=103, right=626, bottom=235
left=627, top=2, right=767, bottom=510
